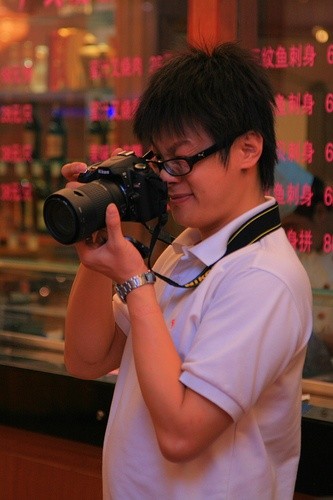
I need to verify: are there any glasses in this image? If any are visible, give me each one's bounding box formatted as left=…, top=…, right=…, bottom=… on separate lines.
left=141, top=134, right=237, bottom=177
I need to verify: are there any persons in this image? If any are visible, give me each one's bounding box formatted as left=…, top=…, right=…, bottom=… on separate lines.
left=61, top=45, right=313, bottom=500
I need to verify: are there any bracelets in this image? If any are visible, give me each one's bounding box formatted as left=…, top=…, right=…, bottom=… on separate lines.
left=114, top=270, right=155, bottom=304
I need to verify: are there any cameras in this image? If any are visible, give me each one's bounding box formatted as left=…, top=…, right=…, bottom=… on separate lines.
left=43, top=151, right=171, bottom=246
